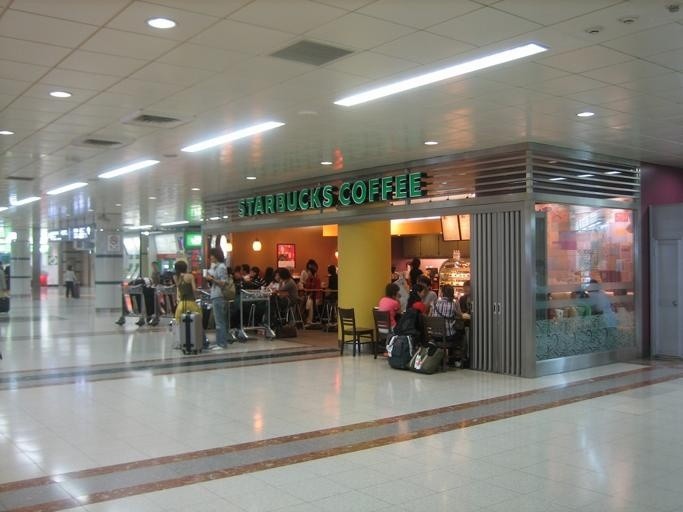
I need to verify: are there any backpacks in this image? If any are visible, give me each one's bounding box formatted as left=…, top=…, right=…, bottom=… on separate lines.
left=393, top=308, right=423, bottom=337
left=385, top=333, right=417, bottom=370
left=453, top=317, right=466, bottom=335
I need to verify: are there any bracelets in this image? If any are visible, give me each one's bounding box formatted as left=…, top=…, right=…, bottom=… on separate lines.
left=209, top=278, right=213, bottom=282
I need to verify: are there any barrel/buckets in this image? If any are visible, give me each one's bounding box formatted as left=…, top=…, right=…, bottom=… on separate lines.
left=599, top=269, right=621, bottom=292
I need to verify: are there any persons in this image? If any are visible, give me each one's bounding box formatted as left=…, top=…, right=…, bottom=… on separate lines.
left=225, top=256, right=338, bottom=334
left=0, top=260, right=7, bottom=298
left=547, top=278, right=630, bottom=329
left=171, top=260, right=202, bottom=325
left=64, top=265, right=78, bottom=298
left=205, top=247, right=229, bottom=353
left=150, top=261, right=160, bottom=286
left=378, top=257, right=472, bottom=368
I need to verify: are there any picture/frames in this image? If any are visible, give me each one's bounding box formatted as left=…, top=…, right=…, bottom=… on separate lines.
left=276, top=243, right=296, bottom=269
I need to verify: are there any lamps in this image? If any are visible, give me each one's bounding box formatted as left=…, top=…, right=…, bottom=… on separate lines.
left=252, top=231, right=262, bottom=252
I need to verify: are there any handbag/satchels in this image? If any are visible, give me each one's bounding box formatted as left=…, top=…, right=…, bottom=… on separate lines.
left=221, top=277, right=236, bottom=301
left=406, top=342, right=445, bottom=374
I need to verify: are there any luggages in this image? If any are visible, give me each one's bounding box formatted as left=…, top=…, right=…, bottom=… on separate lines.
left=180, top=310, right=204, bottom=355
left=128, top=275, right=157, bottom=316
left=0, top=295, right=11, bottom=313
left=72, top=280, right=81, bottom=298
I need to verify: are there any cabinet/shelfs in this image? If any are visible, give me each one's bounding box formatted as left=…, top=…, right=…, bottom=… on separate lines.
left=436, top=257, right=471, bottom=298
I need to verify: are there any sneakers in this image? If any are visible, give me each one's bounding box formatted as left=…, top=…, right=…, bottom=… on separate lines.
left=208, top=343, right=225, bottom=351
left=455, top=359, right=461, bottom=368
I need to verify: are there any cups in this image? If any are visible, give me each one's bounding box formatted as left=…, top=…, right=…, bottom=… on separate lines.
left=202, top=269, right=207, bottom=278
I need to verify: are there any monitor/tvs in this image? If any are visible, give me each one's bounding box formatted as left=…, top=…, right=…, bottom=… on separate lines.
left=426, top=268, right=438, bottom=276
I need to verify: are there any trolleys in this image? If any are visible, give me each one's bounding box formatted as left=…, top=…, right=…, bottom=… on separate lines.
left=228, top=282, right=278, bottom=344
left=115, top=280, right=179, bottom=330
